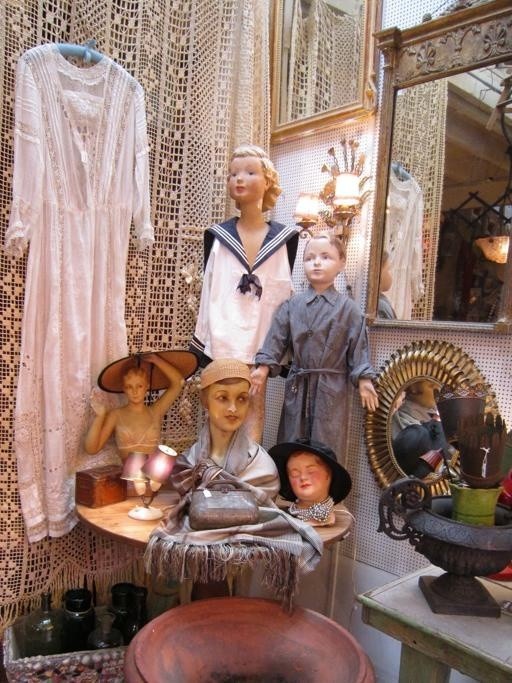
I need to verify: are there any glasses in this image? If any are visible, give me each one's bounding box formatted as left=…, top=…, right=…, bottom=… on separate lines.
left=294, top=139, right=373, bottom=300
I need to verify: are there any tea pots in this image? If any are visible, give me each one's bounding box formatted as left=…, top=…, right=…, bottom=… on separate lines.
left=366, top=2, right=512, bottom=334
left=363, top=340, right=499, bottom=506
left=268, top=0, right=383, bottom=145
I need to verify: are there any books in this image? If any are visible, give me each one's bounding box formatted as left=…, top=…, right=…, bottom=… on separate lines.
left=75, top=465, right=127, bottom=508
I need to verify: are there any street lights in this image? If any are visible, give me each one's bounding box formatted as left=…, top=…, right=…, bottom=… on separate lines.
left=190, top=479, right=260, bottom=531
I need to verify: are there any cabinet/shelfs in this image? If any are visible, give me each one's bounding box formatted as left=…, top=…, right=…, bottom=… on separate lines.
left=20, top=582, right=149, bottom=657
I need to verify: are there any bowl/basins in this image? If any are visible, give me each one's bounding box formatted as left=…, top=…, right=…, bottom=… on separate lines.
left=289, top=495, right=338, bottom=522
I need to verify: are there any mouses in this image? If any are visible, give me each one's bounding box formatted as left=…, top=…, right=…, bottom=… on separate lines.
left=25, top=37, right=138, bottom=91
left=390, top=160, right=422, bottom=195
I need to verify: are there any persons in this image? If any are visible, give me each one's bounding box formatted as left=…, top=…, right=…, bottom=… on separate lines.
left=178, top=357, right=282, bottom=500
left=182, top=145, right=302, bottom=448
left=80, top=354, right=187, bottom=467
left=268, top=439, right=355, bottom=528
left=391, top=380, right=459, bottom=478
left=250, top=235, right=378, bottom=465
left=373, top=249, right=399, bottom=319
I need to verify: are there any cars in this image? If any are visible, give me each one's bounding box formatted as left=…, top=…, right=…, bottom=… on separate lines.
left=446, top=482, right=504, bottom=527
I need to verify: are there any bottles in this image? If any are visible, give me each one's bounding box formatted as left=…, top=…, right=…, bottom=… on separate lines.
left=97, top=346, right=198, bottom=393
left=393, top=421, right=450, bottom=478
left=199, top=358, right=251, bottom=390
left=267, top=436, right=351, bottom=505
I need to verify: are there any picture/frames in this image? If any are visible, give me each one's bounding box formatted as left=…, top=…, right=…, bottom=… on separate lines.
left=357, top=563, right=512, bottom=683
left=76, top=493, right=354, bottom=602
